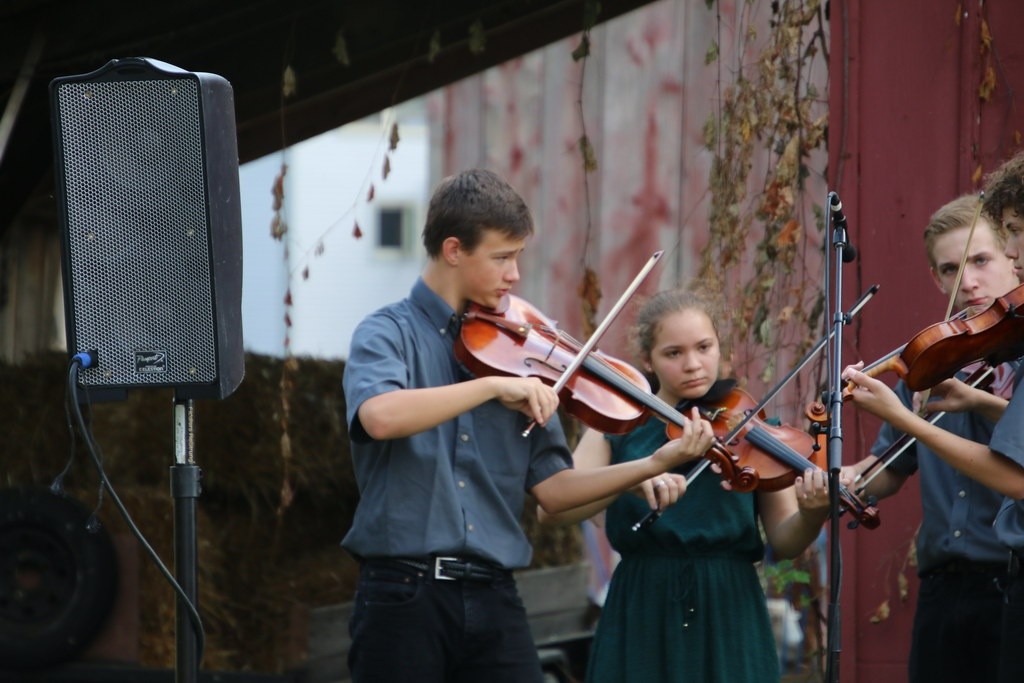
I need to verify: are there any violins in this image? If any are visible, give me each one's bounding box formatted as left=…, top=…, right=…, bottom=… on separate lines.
left=662, top=382, right=884, bottom=531
left=455, top=289, right=760, bottom=494
left=803, top=279, right=1022, bottom=424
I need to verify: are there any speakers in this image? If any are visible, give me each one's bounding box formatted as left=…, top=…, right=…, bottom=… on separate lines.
left=48, top=57, right=246, bottom=404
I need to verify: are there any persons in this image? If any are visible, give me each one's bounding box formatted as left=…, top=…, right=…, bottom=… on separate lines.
left=839, top=156, right=1024, bottom=683
left=536, top=293, right=831, bottom=683
left=339, top=167, right=716, bottom=683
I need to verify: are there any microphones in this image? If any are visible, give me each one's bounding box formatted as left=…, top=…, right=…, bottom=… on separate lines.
left=829, top=191, right=856, bottom=263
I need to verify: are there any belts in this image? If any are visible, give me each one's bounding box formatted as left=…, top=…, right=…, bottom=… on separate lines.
left=366, top=555, right=514, bottom=583
left=1007, top=549, right=1024, bottom=579
left=945, top=556, right=1005, bottom=578
